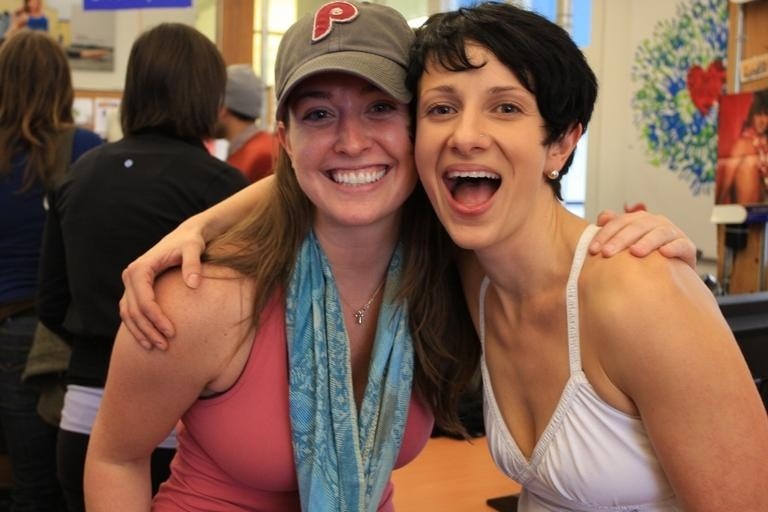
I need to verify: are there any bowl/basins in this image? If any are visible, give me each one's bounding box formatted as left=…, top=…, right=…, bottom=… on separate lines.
left=329, top=277, right=385, bottom=328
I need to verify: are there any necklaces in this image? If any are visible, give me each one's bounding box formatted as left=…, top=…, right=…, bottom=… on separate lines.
left=329, top=277, right=385, bottom=328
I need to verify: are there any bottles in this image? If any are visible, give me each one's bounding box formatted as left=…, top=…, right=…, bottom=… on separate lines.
left=272, top=0, right=417, bottom=119
left=222, top=66, right=265, bottom=120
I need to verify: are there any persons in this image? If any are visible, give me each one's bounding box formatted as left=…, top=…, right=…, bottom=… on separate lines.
left=80, top=0, right=701, bottom=512
left=5, top=0, right=48, bottom=45
left=716, top=92, right=768, bottom=204
left=3, top=34, right=108, bottom=511
left=116, top=0, right=768, bottom=512
left=37, top=23, right=252, bottom=511
left=203, top=63, right=280, bottom=186
